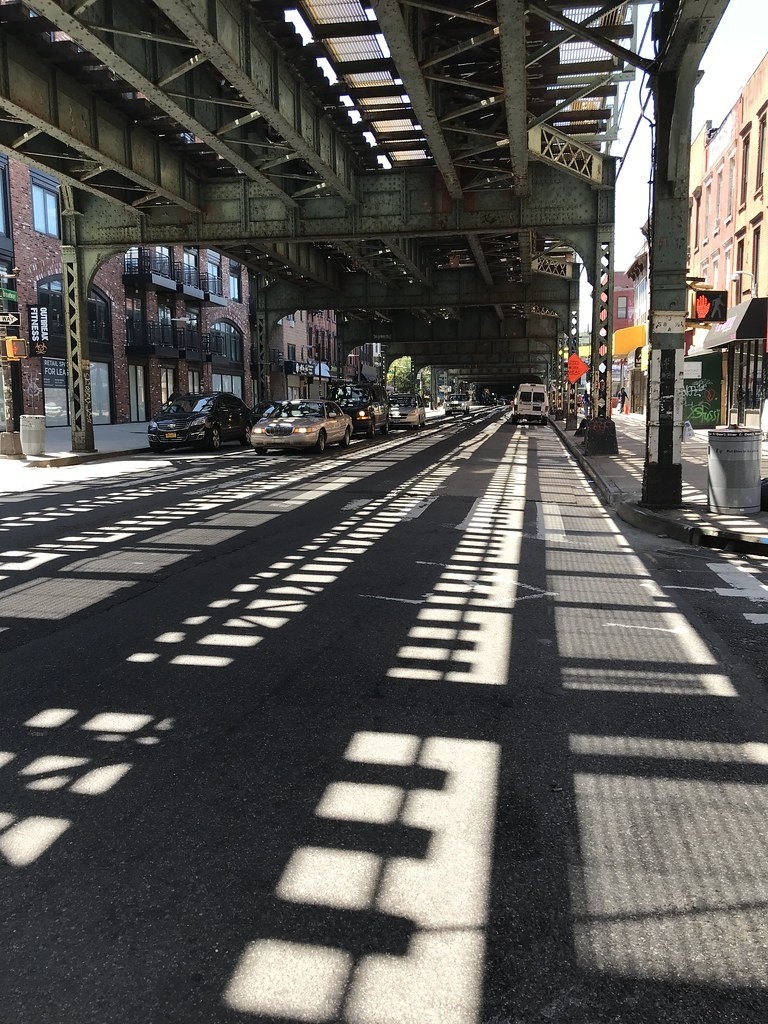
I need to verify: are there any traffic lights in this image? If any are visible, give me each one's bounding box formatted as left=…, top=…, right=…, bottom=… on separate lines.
left=693, top=290, right=729, bottom=323
left=7, top=338, right=27, bottom=360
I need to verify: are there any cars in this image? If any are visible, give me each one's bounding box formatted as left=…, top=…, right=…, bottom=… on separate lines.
left=250, top=398, right=354, bottom=454
left=249, top=400, right=285, bottom=428
left=445, top=394, right=470, bottom=416
left=388, top=393, right=426, bottom=430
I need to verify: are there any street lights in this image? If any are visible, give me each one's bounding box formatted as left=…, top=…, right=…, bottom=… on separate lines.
left=731, top=270, right=755, bottom=297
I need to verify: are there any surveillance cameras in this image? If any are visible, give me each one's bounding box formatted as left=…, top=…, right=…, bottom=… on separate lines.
left=731, top=274, right=740, bottom=282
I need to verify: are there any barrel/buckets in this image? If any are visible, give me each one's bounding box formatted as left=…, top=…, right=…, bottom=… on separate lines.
left=708, top=427, right=762, bottom=514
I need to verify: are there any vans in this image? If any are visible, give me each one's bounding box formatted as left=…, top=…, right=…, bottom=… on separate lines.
left=512, top=383, right=549, bottom=426
left=324, top=382, right=391, bottom=437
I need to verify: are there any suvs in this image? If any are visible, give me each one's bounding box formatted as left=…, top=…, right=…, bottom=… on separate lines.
left=148, top=390, right=254, bottom=451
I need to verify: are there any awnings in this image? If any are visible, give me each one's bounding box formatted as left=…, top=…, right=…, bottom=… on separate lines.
left=702, top=297, right=768, bottom=350
left=612, top=324, right=646, bottom=359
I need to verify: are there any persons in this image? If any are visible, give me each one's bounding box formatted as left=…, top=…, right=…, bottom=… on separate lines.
left=577, top=390, right=590, bottom=410
left=618, top=388, right=629, bottom=414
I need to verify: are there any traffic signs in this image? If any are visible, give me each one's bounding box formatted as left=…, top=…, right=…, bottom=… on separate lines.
left=0, top=312, right=22, bottom=326
